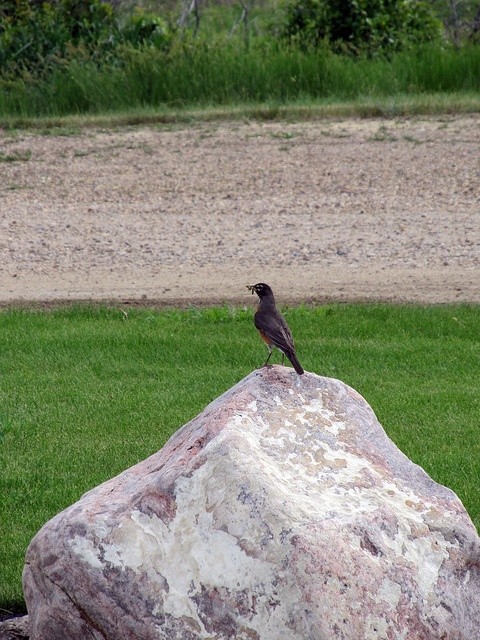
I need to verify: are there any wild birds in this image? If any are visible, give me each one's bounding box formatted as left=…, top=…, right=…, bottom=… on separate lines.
left=245, top=283, right=304, bottom=376
